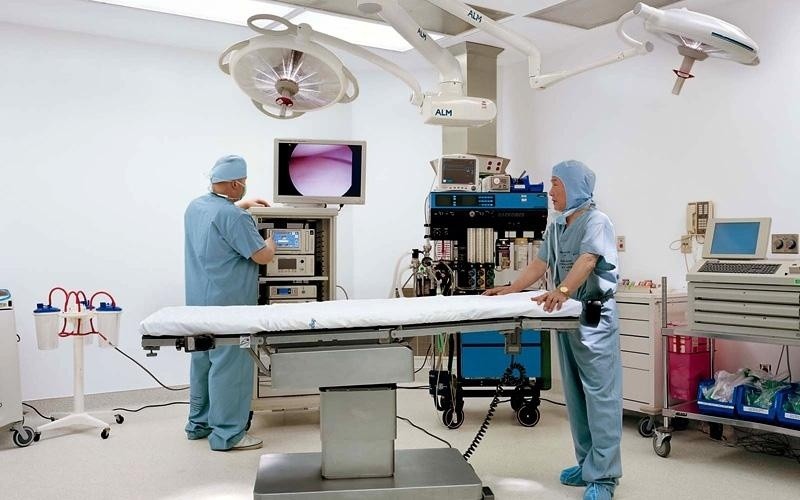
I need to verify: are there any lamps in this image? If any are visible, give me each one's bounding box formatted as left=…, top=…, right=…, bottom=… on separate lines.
left=215, top=0, right=498, bottom=129
left=426, top=0, right=761, bottom=94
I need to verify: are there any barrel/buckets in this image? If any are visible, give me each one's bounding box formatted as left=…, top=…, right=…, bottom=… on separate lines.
left=32, top=303, right=62, bottom=350
left=95, top=302, right=123, bottom=351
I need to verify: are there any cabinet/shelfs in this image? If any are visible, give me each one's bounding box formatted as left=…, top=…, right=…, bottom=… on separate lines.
left=654, top=274, right=800, bottom=458
left=551, top=289, right=688, bottom=418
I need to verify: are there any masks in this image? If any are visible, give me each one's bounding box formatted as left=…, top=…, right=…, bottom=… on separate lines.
left=231, top=179, right=247, bottom=201
left=548, top=200, right=589, bottom=225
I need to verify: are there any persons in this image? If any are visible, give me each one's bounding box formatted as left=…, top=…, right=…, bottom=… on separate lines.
left=183, top=154, right=276, bottom=451
left=484, top=160, right=623, bottom=500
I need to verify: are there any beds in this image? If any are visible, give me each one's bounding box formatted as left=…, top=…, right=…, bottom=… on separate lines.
left=140, top=288, right=584, bottom=500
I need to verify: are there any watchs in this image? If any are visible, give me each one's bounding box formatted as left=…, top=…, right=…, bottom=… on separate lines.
left=556, top=286, right=570, bottom=299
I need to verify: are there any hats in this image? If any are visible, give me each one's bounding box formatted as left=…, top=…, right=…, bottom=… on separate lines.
left=551, top=160, right=595, bottom=211
left=210, top=154, right=248, bottom=184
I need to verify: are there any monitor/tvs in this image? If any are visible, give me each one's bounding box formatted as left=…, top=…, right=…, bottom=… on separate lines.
left=272, top=137, right=368, bottom=204
left=702, top=217, right=772, bottom=259
left=439, top=154, right=479, bottom=192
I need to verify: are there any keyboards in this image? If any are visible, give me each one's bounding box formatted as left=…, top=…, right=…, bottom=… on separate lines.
left=697, top=261, right=782, bottom=274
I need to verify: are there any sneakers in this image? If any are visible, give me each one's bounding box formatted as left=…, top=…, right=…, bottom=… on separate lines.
left=560, top=466, right=587, bottom=487
left=583, top=482, right=614, bottom=500
left=232, top=433, right=264, bottom=451
